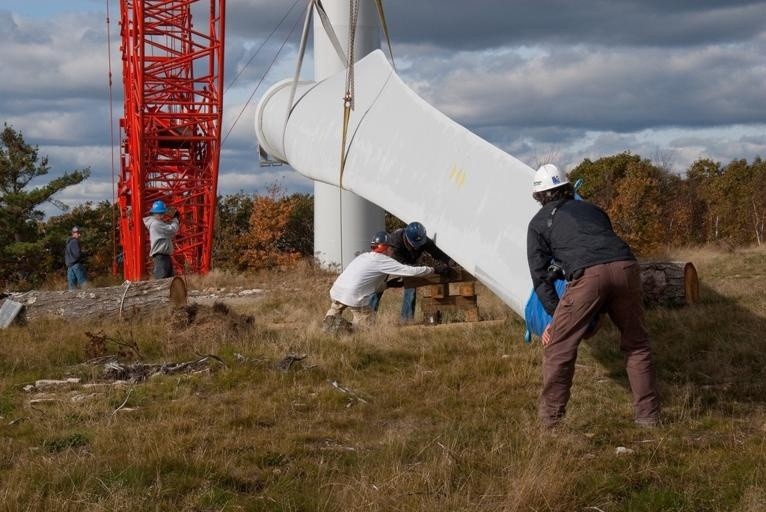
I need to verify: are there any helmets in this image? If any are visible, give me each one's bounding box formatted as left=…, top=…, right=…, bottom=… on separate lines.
left=370, top=230, right=395, bottom=247
left=150, top=200, right=169, bottom=213
left=72, top=226, right=80, bottom=232
left=532, top=163, right=570, bottom=193
left=404, top=221, right=427, bottom=248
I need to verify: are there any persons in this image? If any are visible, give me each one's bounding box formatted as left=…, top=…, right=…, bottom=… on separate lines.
left=63, top=226, right=88, bottom=292
left=368, top=222, right=450, bottom=323
left=322, top=231, right=457, bottom=338
left=527, top=163, right=664, bottom=434
left=142, top=201, right=180, bottom=279
